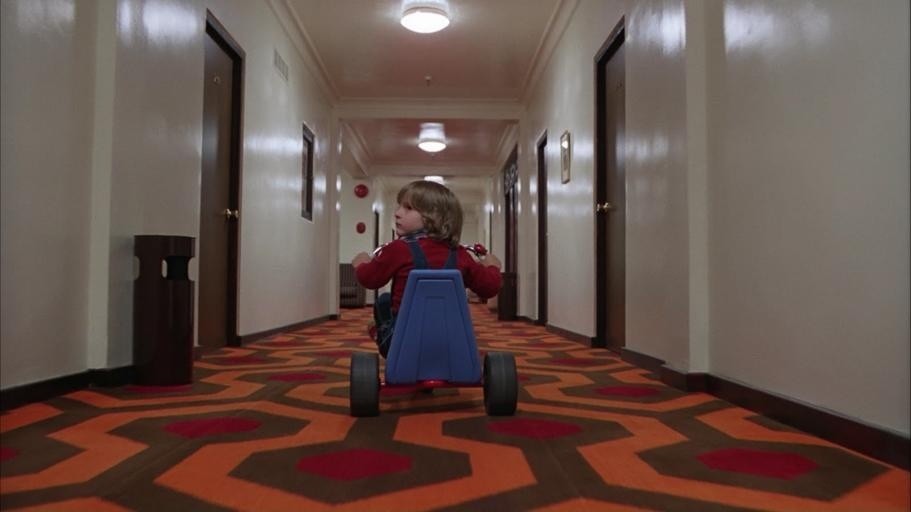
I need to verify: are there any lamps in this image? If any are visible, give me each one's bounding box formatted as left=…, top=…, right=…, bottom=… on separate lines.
left=395, top=0, right=452, bottom=37
left=422, top=175, right=447, bottom=187
left=416, top=120, right=450, bottom=153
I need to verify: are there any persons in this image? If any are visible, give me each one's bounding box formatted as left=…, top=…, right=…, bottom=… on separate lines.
left=349, top=178, right=503, bottom=357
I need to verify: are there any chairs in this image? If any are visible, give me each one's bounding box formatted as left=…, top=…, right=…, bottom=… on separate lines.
left=337, top=262, right=369, bottom=309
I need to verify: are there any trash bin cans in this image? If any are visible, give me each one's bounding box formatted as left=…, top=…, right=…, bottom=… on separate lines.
left=130, top=232, right=199, bottom=387
left=496, top=271, right=517, bottom=322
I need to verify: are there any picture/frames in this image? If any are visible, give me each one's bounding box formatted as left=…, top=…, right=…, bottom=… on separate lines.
left=559, top=129, right=572, bottom=185
